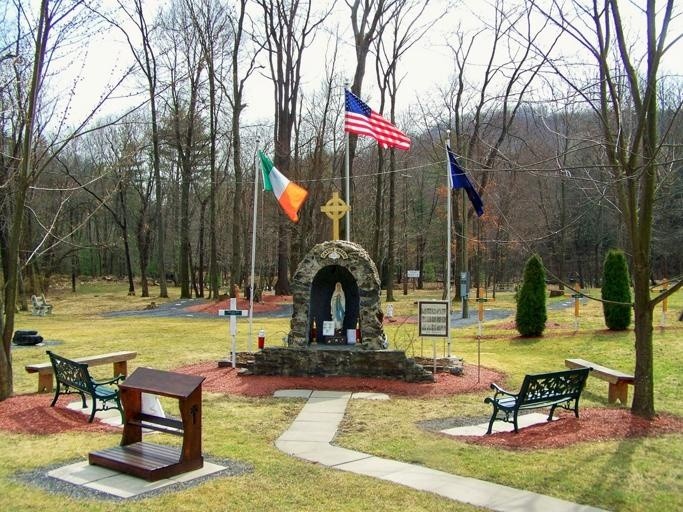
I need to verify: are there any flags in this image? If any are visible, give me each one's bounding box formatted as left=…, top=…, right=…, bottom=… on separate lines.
left=445, top=145, right=484, bottom=217
left=257, top=149, right=308, bottom=222
left=344, top=87, right=411, bottom=151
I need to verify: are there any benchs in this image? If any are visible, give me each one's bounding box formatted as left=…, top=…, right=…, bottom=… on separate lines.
left=486, top=358, right=634, bottom=434
left=26, top=351, right=136, bottom=424
